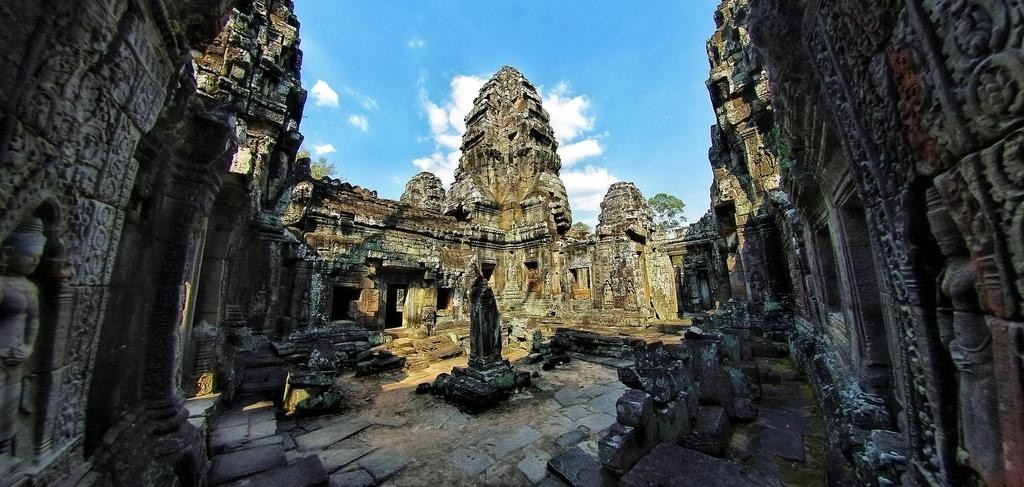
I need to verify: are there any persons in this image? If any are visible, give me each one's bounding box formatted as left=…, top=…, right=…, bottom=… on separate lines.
left=477, top=277, right=498, bottom=357
left=468, top=274, right=484, bottom=353
left=926, top=188, right=1008, bottom=486
left=0, top=214, right=47, bottom=453
